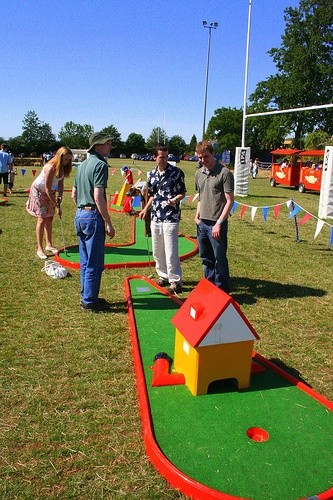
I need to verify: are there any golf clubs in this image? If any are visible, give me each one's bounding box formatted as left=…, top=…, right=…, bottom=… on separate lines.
left=146, top=234, right=154, bottom=279
left=57, top=195, right=70, bottom=257
left=132, top=161, right=147, bottom=177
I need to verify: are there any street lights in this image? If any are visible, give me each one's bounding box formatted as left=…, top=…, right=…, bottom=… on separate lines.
left=202, top=20, right=218, bottom=139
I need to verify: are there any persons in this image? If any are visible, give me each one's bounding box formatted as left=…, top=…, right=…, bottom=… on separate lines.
left=130, top=178, right=149, bottom=200
left=280, top=156, right=324, bottom=173
left=8, top=163, right=17, bottom=194
left=194, top=141, right=234, bottom=294
left=71, top=132, right=114, bottom=310
left=0, top=143, right=12, bottom=196
left=122, top=166, right=133, bottom=187
left=250, top=158, right=259, bottom=179
left=30, top=149, right=86, bottom=165
left=139, top=146, right=187, bottom=293
left=26, top=147, right=73, bottom=259
left=123, top=191, right=134, bottom=216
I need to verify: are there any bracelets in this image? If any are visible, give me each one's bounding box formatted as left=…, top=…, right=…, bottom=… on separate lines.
left=175, top=197, right=179, bottom=203
left=217, top=219, right=223, bottom=225
left=59, top=191, right=63, bottom=193
left=146, top=204, right=150, bottom=209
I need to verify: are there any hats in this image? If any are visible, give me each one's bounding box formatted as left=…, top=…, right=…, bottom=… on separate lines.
left=122, top=166, right=128, bottom=170
left=88, top=131, right=115, bottom=152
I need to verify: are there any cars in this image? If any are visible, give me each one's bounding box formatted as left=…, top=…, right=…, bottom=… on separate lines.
left=131, top=153, right=154, bottom=160
left=168, top=153, right=198, bottom=161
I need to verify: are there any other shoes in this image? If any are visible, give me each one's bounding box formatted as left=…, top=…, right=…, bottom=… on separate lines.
left=3, top=193, right=7, bottom=197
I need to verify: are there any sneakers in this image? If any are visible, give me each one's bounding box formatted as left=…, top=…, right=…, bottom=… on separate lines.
left=37, top=251, right=47, bottom=259
left=79, top=298, right=110, bottom=311
left=156, top=279, right=170, bottom=287
left=46, top=246, right=58, bottom=253
left=167, top=282, right=182, bottom=295
